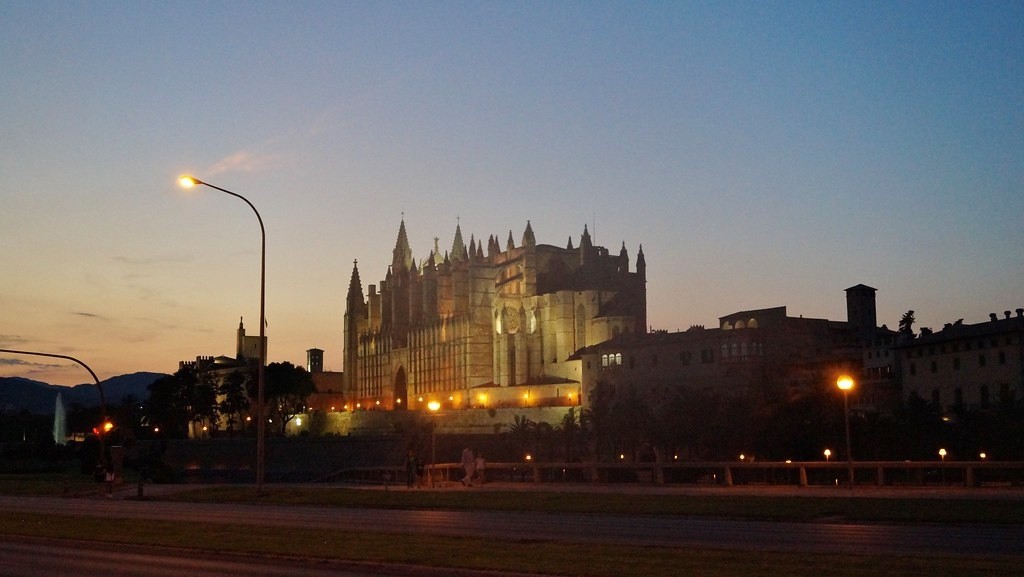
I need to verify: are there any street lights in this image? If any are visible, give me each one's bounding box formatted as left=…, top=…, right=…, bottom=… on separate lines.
left=376, top=401, right=380, bottom=411
left=357, top=403, right=360, bottom=412
left=331, top=406, right=335, bottom=413
left=674, top=455, right=680, bottom=483
left=836, top=373, right=857, bottom=488
left=938, top=449, right=947, bottom=487
left=203, top=426, right=207, bottom=439
left=568, top=393, right=572, bottom=405
left=740, top=455, right=745, bottom=485
left=296, top=419, right=301, bottom=435
left=481, top=396, right=485, bottom=408
left=524, top=394, right=529, bottom=408
left=154, top=427, right=159, bottom=439
left=449, top=397, right=452, bottom=409
left=177, top=171, right=267, bottom=498
left=979, top=453, right=987, bottom=487
left=620, top=454, right=626, bottom=482
left=824, top=448, right=831, bottom=485
left=397, top=399, right=401, bottom=409
left=525, top=454, right=538, bottom=482
left=419, top=397, right=423, bottom=411
left=344, top=405, right=348, bottom=412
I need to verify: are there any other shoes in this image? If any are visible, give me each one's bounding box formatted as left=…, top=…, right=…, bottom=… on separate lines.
left=468, top=483, right=473, bottom=487
left=459, top=480, right=466, bottom=486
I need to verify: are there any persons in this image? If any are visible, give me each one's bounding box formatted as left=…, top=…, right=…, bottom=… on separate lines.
left=460, top=445, right=475, bottom=487
left=136, top=467, right=146, bottom=496
left=404, top=451, right=414, bottom=488
left=414, top=458, right=423, bottom=489
left=93, top=460, right=115, bottom=497
left=475, top=453, right=485, bottom=484
